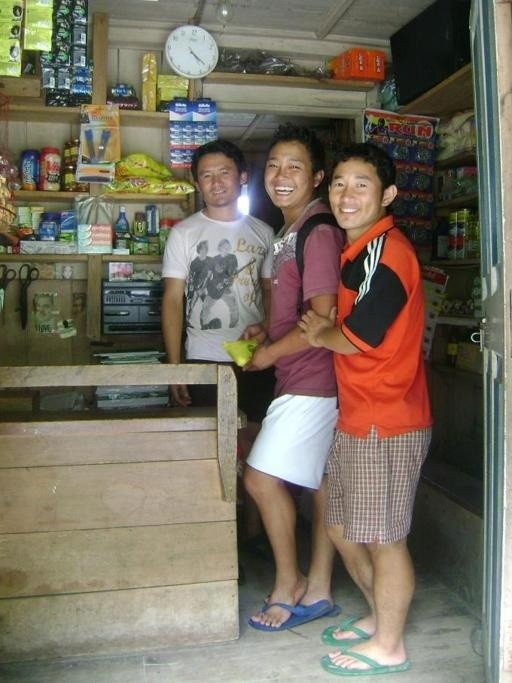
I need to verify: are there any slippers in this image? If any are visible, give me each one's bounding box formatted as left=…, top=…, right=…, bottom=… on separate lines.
left=248, top=599, right=332, bottom=629
left=321, top=646, right=410, bottom=676
left=322, top=615, right=373, bottom=644
left=263, top=593, right=341, bottom=616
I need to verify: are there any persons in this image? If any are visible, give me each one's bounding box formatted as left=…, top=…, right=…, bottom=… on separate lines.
left=162, top=139, right=276, bottom=554
left=184, top=242, right=214, bottom=328
left=199, top=240, right=239, bottom=329
left=297, top=143, right=434, bottom=675
left=239, top=122, right=346, bottom=631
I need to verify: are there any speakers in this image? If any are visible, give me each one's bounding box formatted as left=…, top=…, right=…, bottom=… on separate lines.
left=389, top=0, right=471, bottom=105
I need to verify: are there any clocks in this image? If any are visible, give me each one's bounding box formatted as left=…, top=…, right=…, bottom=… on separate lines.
left=163, top=22, right=223, bottom=80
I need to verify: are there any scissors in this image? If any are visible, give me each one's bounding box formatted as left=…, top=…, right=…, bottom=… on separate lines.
left=19, top=264, right=39, bottom=330
left=0, top=264, right=17, bottom=311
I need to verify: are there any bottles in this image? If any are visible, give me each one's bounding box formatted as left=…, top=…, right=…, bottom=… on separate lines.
left=429, top=207, right=480, bottom=260
left=446, top=323, right=458, bottom=367
left=114, top=204, right=159, bottom=250
left=38, top=212, right=60, bottom=240
left=19, top=138, right=89, bottom=191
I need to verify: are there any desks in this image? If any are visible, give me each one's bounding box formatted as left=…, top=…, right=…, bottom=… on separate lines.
left=0, top=402, right=250, bottom=665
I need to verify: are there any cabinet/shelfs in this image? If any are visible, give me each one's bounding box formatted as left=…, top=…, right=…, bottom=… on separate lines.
left=395, top=61, right=483, bottom=521
left=1, top=7, right=202, bottom=346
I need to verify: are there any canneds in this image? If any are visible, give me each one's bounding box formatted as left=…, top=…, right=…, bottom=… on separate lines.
left=21, top=138, right=90, bottom=191
